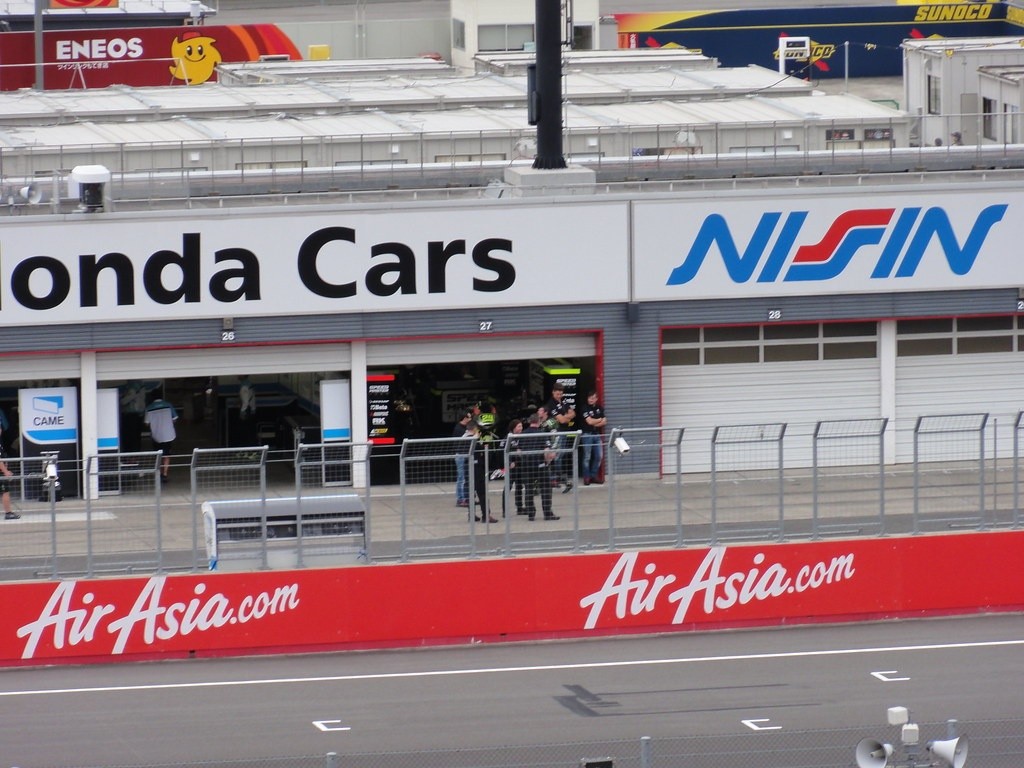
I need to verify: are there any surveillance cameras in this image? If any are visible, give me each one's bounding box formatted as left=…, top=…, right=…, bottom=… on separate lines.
left=46, top=464, right=57, bottom=481
left=615, top=437, right=630, bottom=454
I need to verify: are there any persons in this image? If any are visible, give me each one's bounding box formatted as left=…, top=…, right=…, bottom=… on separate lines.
left=453, top=383, right=607, bottom=523
left=144, top=388, right=179, bottom=483
left=0, top=425, right=21, bottom=519
left=235, top=376, right=260, bottom=460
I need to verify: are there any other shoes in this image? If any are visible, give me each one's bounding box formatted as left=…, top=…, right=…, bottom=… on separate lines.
left=544, top=514, right=561, bottom=521
left=456, top=501, right=470, bottom=507
left=481, top=514, right=498, bottom=523
left=584, top=476, right=590, bottom=485
left=162, top=475, right=170, bottom=483
left=590, top=477, right=604, bottom=484
left=562, top=482, right=573, bottom=494
left=467, top=513, right=480, bottom=521
left=5, top=511, right=21, bottom=520
left=517, top=508, right=527, bottom=515
left=528, top=516, right=535, bottom=521
left=552, top=479, right=560, bottom=488
